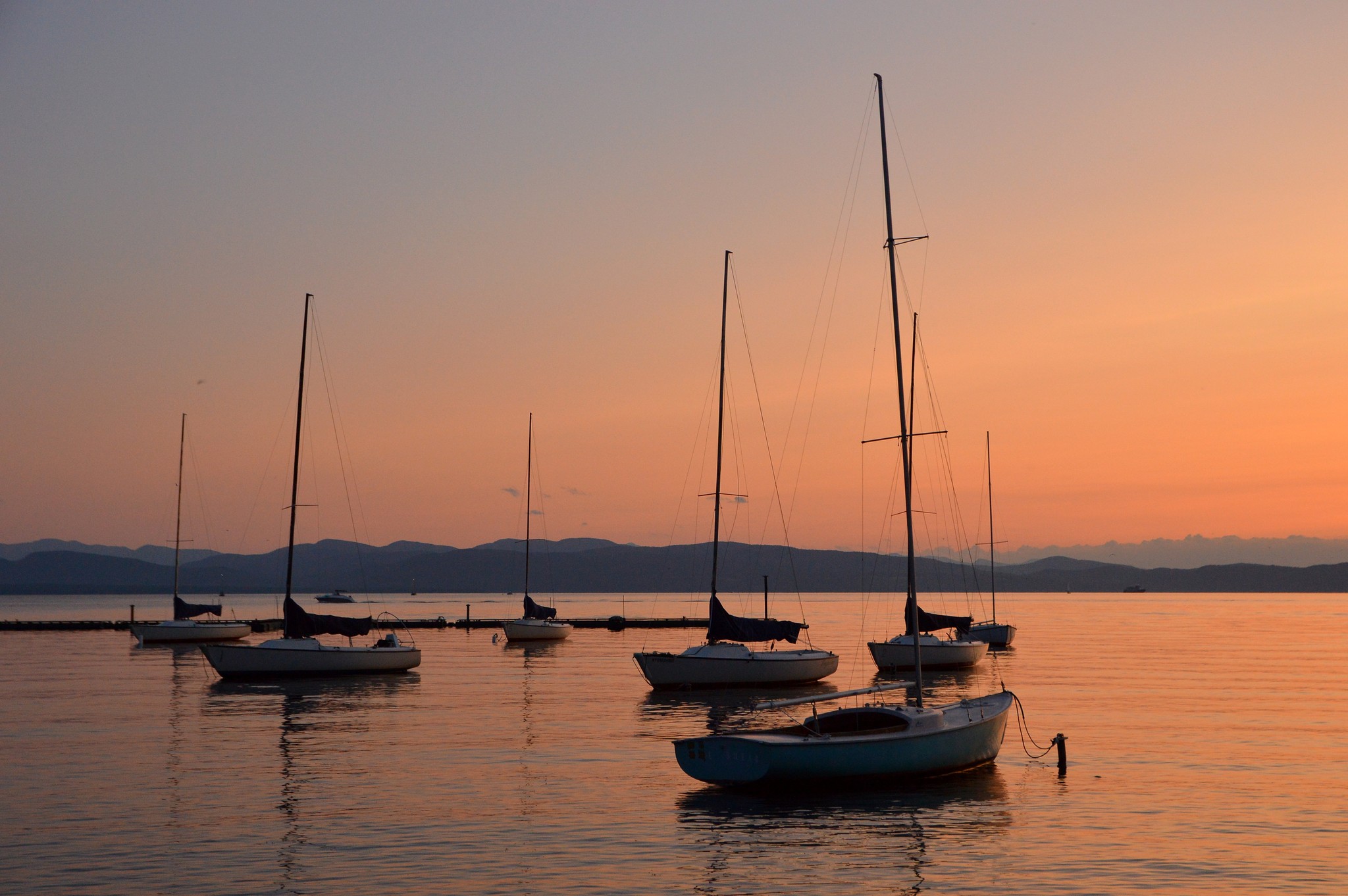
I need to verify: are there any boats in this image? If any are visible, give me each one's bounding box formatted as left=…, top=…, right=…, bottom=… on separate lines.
left=280, top=597, right=371, bottom=638
left=313, top=589, right=356, bottom=605
left=707, top=575, right=809, bottom=645
left=903, top=593, right=974, bottom=634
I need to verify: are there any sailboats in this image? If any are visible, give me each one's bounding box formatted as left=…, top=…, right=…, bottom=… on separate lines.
left=967, top=430, right=1017, bottom=646
left=501, top=412, right=575, bottom=641
left=198, top=293, right=421, bottom=681
left=125, top=412, right=253, bottom=645
left=660, top=309, right=1015, bottom=793
left=865, top=72, right=991, bottom=673
left=630, top=250, right=839, bottom=688
